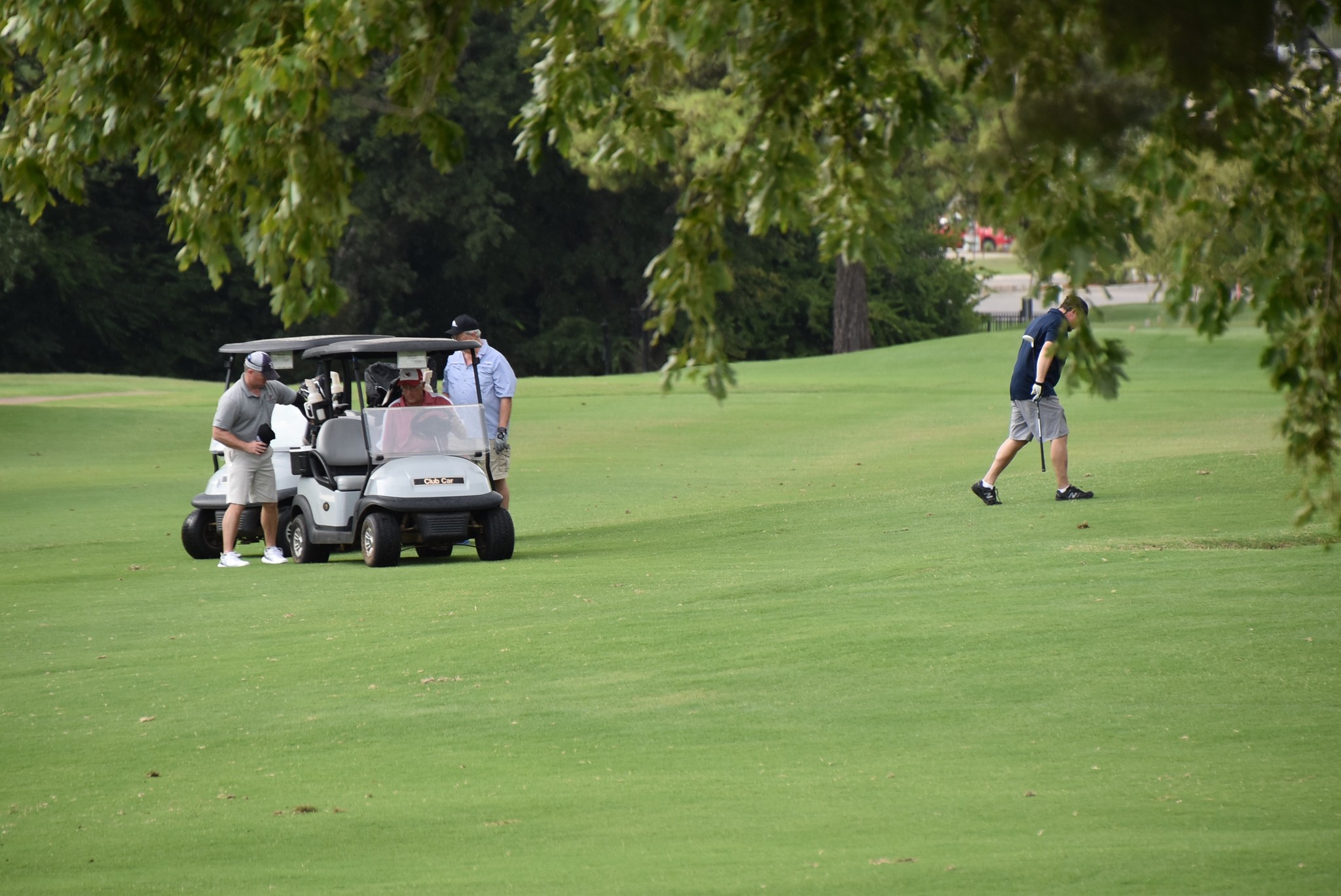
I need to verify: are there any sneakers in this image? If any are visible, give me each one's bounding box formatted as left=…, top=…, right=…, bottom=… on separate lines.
left=217, top=552, right=250, bottom=568
left=1055, top=484, right=1094, bottom=501
left=972, top=480, right=1002, bottom=506
left=261, top=547, right=288, bottom=564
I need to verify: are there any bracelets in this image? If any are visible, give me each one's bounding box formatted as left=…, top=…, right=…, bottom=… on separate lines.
left=497, top=427, right=507, bottom=433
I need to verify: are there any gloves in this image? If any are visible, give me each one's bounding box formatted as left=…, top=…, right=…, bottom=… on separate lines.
left=1031, top=382, right=1044, bottom=404
left=494, top=432, right=510, bottom=455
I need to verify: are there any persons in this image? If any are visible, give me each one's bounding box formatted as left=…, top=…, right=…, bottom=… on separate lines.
left=378, top=314, right=518, bottom=510
left=972, top=295, right=1094, bottom=506
left=212, top=351, right=310, bottom=567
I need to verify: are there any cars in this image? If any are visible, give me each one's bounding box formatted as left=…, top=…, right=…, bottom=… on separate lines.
left=934, top=211, right=1017, bottom=252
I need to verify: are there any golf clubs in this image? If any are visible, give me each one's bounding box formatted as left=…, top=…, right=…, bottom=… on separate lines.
left=1022, top=334, right=1047, bottom=472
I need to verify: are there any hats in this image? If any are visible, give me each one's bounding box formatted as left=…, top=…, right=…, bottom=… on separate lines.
left=397, top=369, right=423, bottom=387
left=245, top=351, right=280, bottom=380
left=445, top=315, right=479, bottom=334
left=1065, top=295, right=1088, bottom=323
